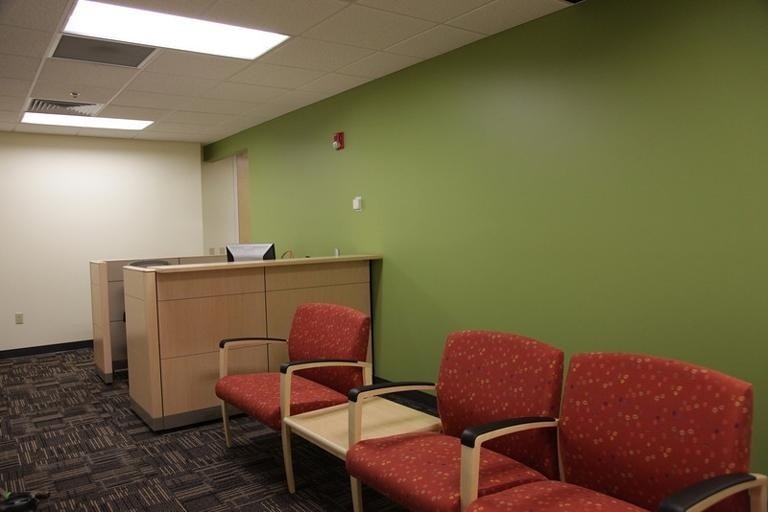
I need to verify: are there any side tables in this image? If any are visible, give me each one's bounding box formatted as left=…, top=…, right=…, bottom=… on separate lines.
left=283, top=395, right=444, bottom=511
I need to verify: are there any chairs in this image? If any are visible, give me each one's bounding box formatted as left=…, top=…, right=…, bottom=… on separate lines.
left=214, top=302, right=372, bottom=495
left=460, top=350, right=767, bottom=512
left=349, top=329, right=564, bottom=511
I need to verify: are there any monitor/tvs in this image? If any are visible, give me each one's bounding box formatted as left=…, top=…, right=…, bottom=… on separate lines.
left=226, top=243, right=275, bottom=262
